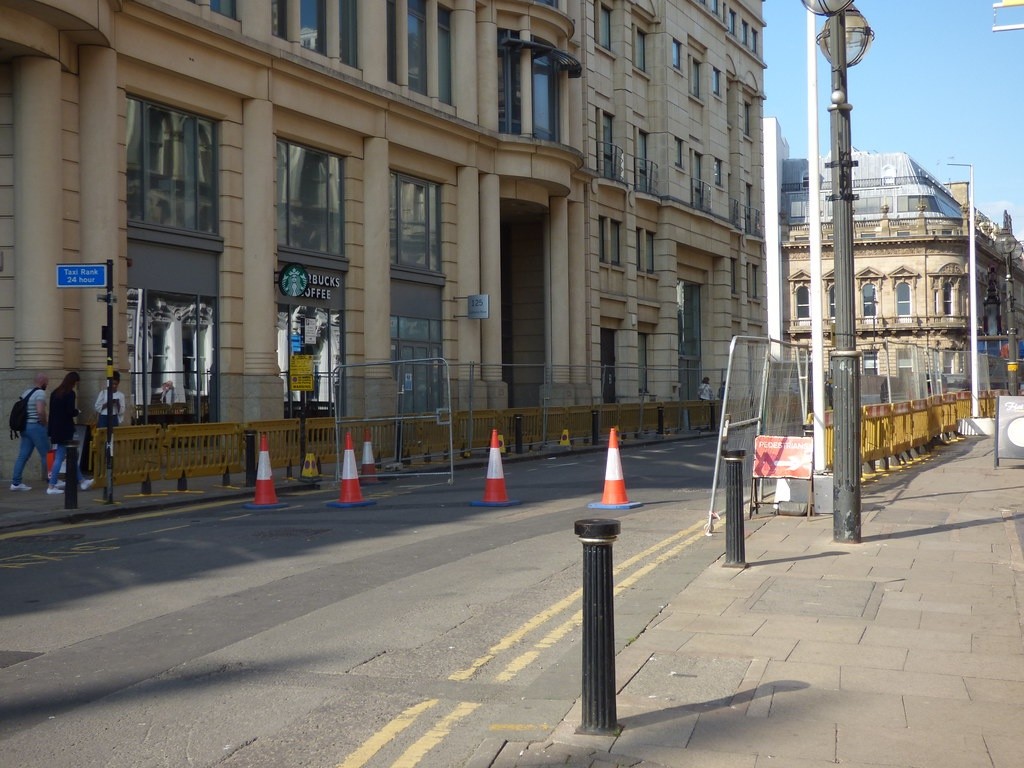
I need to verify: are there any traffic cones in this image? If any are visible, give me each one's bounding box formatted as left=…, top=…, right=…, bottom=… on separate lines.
left=472, top=428, right=522, bottom=507
left=242, top=435, right=291, bottom=510
left=585, top=427, right=642, bottom=510
left=498, top=434, right=510, bottom=458
left=560, top=429, right=571, bottom=446
left=358, top=426, right=385, bottom=486
left=297, top=452, right=323, bottom=483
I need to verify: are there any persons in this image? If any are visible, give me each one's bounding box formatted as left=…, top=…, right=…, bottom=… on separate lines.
left=718, top=381, right=729, bottom=399
left=697, top=376, right=713, bottom=400
left=159, top=381, right=180, bottom=403
left=93, top=371, right=125, bottom=469
left=47, top=371, right=92, bottom=494
left=9, top=372, right=51, bottom=491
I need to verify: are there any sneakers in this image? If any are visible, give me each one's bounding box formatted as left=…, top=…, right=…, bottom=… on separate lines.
left=80, top=479, right=94, bottom=490
left=46, top=486, right=64, bottom=495
left=55, top=480, right=66, bottom=487
left=10, top=482, right=32, bottom=492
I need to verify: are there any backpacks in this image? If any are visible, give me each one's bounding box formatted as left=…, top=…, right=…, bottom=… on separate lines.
left=9, top=387, right=40, bottom=432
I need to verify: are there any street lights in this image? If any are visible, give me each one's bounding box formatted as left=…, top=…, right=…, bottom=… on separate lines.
left=948, top=163, right=979, bottom=418
left=801, top=0, right=877, bottom=545
left=995, top=210, right=1023, bottom=398
